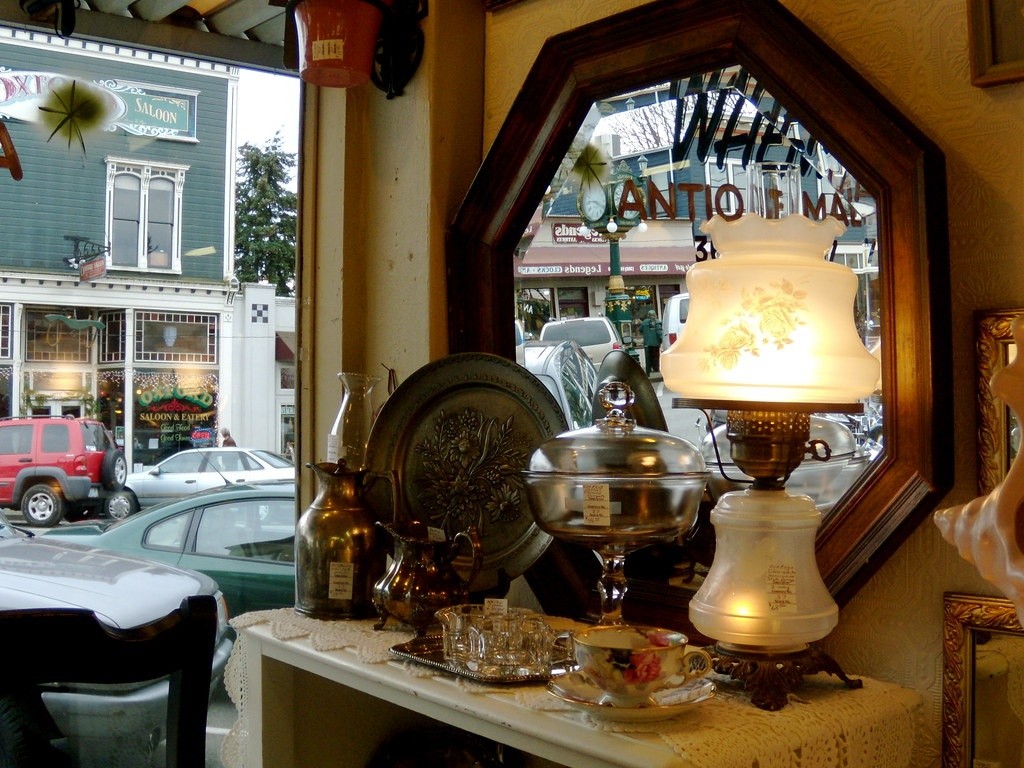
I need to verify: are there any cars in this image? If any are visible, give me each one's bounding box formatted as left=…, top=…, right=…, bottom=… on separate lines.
left=104, top=445, right=295, bottom=518
left=0, top=514, right=234, bottom=760
left=705, top=410, right=861, bottom=435
left=524, top=341, right=596, bottom=432
left=39, top=481, right=297, bottom=613
left=515, top=319, right=532, bottom=368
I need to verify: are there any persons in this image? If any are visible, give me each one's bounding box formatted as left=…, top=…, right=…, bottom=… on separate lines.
left=639, top=311, right=662, bottom=371
left=219, top=428, right=239, bottom=471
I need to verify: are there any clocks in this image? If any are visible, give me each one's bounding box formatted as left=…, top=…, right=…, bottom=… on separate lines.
left=613, top=184, right=643, bottom=218
left=584, top=186, right=608, bottom=222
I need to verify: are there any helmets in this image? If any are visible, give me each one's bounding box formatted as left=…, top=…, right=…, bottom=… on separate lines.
left=648, top=310, right=656, bottom=316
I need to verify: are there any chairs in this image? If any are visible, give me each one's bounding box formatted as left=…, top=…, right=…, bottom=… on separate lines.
left=0, top=594, right=218, bottom=768
left=209, top=452, right=236, bottom=472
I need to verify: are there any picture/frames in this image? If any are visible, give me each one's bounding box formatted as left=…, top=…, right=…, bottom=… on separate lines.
left=943, top=591, right=1024, bottom=768
left=971, top=309, right=1024, bottom=494
left=966, top=0, right=1024, bottom=86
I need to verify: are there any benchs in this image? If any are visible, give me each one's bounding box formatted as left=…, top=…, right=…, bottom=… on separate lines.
left=196, top=527, right=295, bottom=562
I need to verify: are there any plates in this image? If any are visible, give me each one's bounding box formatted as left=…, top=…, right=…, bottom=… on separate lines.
left=364, top=353, right=570, bottom=581
left=544, top=670, right=717, bottom=723
left=591, top=351, right=670, bottom=433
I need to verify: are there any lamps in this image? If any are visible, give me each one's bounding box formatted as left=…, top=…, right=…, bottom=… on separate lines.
left=164, top=326, right=178, bottom=346
left=661, top=213, right=881, bottom=709
left=291, top=1, right=429, bottom=100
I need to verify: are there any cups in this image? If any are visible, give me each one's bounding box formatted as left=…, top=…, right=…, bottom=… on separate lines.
left=435, top=602, right=574, bottom=676
left=573, top=626, right=712, bottom=707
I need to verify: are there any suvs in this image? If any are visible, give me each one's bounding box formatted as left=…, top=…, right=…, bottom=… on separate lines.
left=538, top=312, right=625, bottom=375
left=0, top=416, right=129, bottom=526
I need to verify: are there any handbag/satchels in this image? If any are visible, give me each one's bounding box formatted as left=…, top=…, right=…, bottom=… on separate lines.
left=655, top=320, right=662, bottom=344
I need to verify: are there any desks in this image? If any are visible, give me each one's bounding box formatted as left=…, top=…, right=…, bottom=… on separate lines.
left=223, top=609, right=924, bottom=768
left=149, top=251, right=169, bottom=267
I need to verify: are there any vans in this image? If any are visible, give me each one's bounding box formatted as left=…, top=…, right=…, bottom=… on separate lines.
left=661, top=292, right=689, bottom=351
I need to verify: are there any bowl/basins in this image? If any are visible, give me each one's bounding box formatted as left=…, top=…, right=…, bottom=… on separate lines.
left=701, top=415, right=868, bottom=513
left=518, top=381, right=712, bottom=546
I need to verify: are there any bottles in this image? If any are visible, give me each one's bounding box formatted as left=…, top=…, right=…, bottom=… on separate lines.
left=327, top=372, right=380, bottom=472
left=294, top=458, right=386, bottom=620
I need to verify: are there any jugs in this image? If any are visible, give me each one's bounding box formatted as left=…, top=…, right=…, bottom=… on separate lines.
left=376, top=518, right=483, bottom=638
